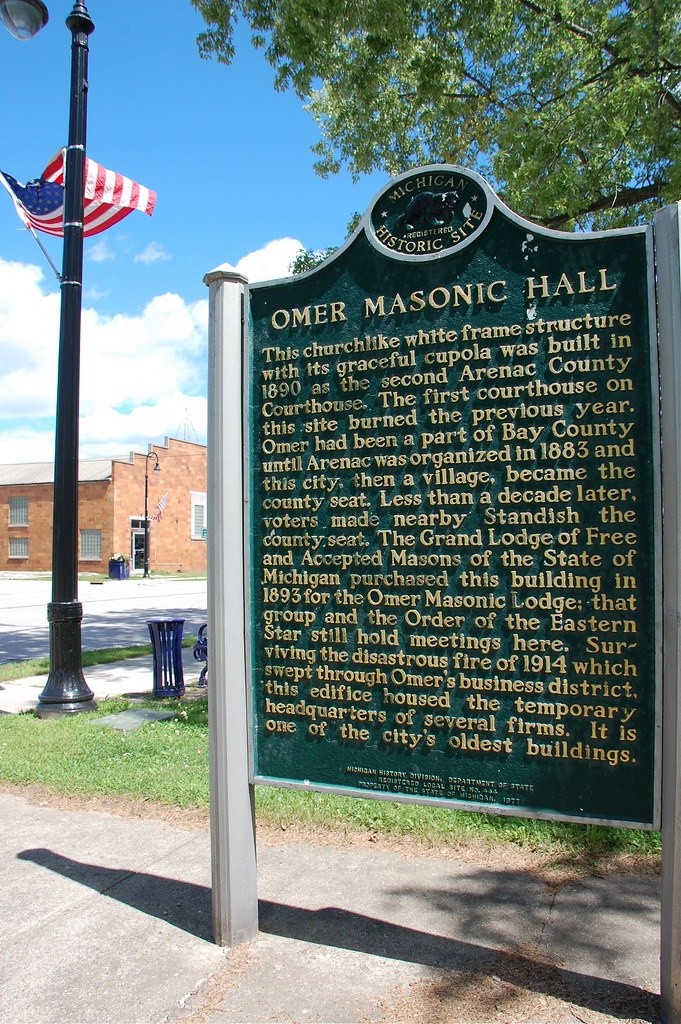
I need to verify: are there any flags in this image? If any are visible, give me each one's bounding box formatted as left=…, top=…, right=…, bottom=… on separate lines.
left=147, top=495, right=166, bottom=522
left=0, top=149, right=157, bottom=239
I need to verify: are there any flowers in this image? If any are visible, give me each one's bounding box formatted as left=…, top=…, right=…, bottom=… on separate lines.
left=108, top=552, right=130, bottom=562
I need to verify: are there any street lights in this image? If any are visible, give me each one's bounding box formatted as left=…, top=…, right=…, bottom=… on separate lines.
left=143, top=452, right=162, bottom=578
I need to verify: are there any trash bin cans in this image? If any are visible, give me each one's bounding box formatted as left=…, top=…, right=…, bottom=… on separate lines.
left=144, top=612, right=188, bottom=697
left=108, top=558, right=130, bottom=580
